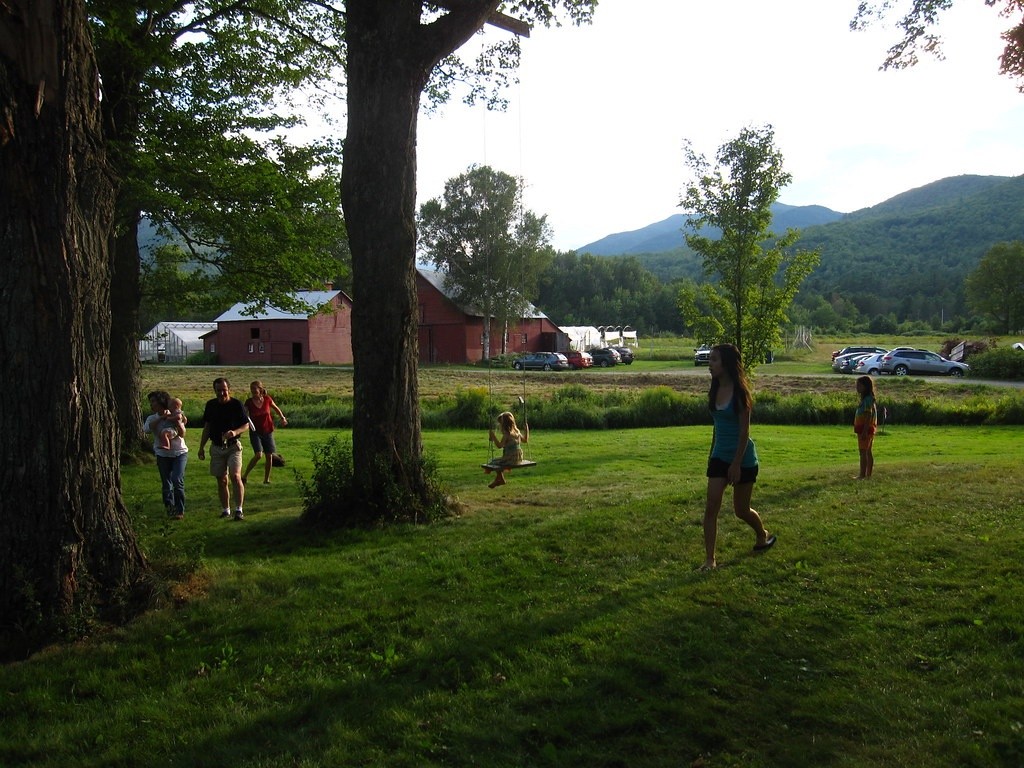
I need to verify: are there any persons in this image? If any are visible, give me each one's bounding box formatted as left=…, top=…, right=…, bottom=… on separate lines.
left=281, top=416, right=286, bottom=419
left=142, top=391, right=189, bottom=520
left=156, top=397, right=182, bottom=450
left=692, top=344, right=778, bottom=573
left=853, top=376, right=877, bottom=479
left=241, top=380, right=288, bottom=485
left=198, top=377, right=250, bottom=521
left=484, top=411, right=529, bottom=488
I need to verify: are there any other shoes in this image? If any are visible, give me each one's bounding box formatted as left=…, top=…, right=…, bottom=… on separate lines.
left=263, top=481, right=271, bottom=484
left=242, top=478, right=246, bottom=483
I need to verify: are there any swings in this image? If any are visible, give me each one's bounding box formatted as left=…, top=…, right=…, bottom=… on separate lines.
left=480, top=23, right=537, bottom=472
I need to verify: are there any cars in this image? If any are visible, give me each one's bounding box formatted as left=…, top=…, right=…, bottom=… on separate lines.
left=890, top=346, right=917, bottom=353
left=857, top=354, right=893, bottom=376
left=512, top=352, right=569, bottom=372
left=561, top=352, right=593, bottom=370
left=833, top=351, right=873, bottom=370
left=830, top=351, right=840, bottom=362
left=842, top=355, right=873, bottom=373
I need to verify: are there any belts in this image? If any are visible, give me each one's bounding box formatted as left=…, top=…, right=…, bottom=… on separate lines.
left=212, top=439, right=239, bottom=447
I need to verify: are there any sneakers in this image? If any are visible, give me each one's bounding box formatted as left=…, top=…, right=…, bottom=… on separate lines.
left=235, top=511, right=243, bottom=520
left=219, top=512, right=231, bottom=519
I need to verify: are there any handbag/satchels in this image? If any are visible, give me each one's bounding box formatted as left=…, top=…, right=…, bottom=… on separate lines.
left=254, top=413, right=274, bottom=435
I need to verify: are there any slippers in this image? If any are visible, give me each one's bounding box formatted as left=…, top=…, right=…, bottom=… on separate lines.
left=754, top=536, right=776, bottom=549
left=699, top=564, right=714, bottom=574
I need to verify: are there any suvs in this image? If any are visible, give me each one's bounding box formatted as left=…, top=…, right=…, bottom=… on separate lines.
left=882, top=346, right=971, bottom=380
left=615, top=346, right=635, bottom=366
left=588, top=347, right=623, bottom=367
left=694, top=344, right=714, bottom=365
left=839, top=347, right=889, bottom=356
